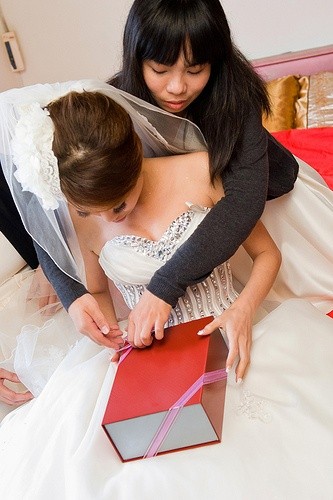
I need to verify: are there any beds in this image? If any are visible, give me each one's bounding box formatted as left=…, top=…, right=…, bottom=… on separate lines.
left=0, top=43, right=333, bottom=499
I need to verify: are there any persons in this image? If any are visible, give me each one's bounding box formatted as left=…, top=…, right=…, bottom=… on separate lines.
left=0, top=164, right=63, bottom=408
left=26, top=0, right=332, bottom=347
left=0, top=78, right=333, bottom=500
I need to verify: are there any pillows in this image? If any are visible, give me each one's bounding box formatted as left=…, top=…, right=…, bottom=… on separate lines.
left=307, top=72, right=333, bottom=128
left=261, top=72, right=309, bottom=134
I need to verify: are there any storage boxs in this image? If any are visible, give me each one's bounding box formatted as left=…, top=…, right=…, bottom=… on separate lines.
left=101, top=317, right=230, bottom=463
